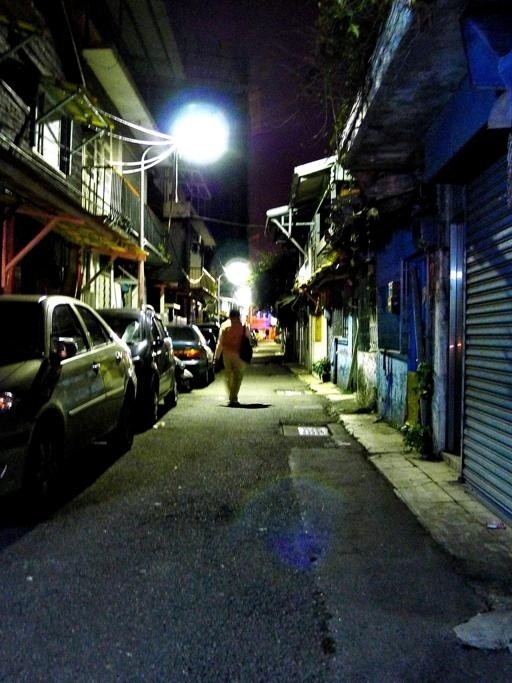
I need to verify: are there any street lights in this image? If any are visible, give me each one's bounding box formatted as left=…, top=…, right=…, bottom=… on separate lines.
left=137, top=100, right=230, bottom=305
left=217, top=257, right=253, bottom=325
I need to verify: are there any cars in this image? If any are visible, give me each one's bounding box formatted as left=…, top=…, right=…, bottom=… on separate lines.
left=197, top=323, right=225, bottom=374
left=164, top=320, right=216, bottom=387
left=251, top=329, right=258, bottom=347
left=0, top=291, right=139, bottom=489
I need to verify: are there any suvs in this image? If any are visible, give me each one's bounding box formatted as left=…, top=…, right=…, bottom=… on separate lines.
left=88, top=302, right=179, bottom=426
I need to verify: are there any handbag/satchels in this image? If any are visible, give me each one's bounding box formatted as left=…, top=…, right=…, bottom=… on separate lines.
left=240, top=336, right=253, bottom=362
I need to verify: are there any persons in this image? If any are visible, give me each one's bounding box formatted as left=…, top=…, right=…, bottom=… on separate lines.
left=231, top=404, right=270, bottom=410
left=214, top=310, right=252, bottom=405
